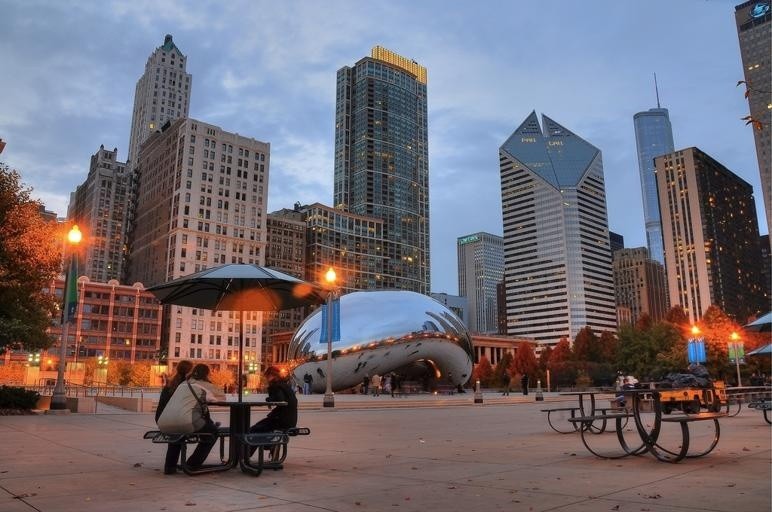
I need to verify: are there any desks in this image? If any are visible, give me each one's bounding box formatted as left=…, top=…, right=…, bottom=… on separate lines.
left=208, top=400, right=288, bottom=477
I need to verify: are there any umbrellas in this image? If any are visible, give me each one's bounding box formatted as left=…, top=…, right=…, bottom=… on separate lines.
left=741, top=313, right=772, bottom=332
left=745, top=343, right=772, bottom=357
left=145, top=260, right=327, bottom=401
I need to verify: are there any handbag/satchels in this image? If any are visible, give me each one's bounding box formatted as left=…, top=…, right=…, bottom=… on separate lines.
left=200, top=403, right=213, bottom=424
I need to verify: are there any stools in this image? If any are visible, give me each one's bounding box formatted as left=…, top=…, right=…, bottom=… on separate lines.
left=142, top=426, right=311, bottom=477
left=539, top=387, right=772, bottom=467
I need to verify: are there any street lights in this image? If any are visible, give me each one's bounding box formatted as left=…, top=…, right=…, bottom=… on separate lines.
left=691, top=325, right=700, bottom=366
left=323, top=266, right=335, bottom=407
left=49, top=225, right=83, bottom=410
left=730, top=332, right=743, bottom=387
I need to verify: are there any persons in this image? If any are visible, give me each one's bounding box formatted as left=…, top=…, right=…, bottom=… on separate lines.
left=501, top=369, right=511, bottom=396
left=520, top=372, right=529, bottom=394
left=154, top=360, right=194, bottom=424
left=614, top=378, right=635, bottom=407
left=156, top=363, right=228, bottom=475
left=241, top=365, right=300, bottom=459
left=222, top=370, right=482, bottom=398
